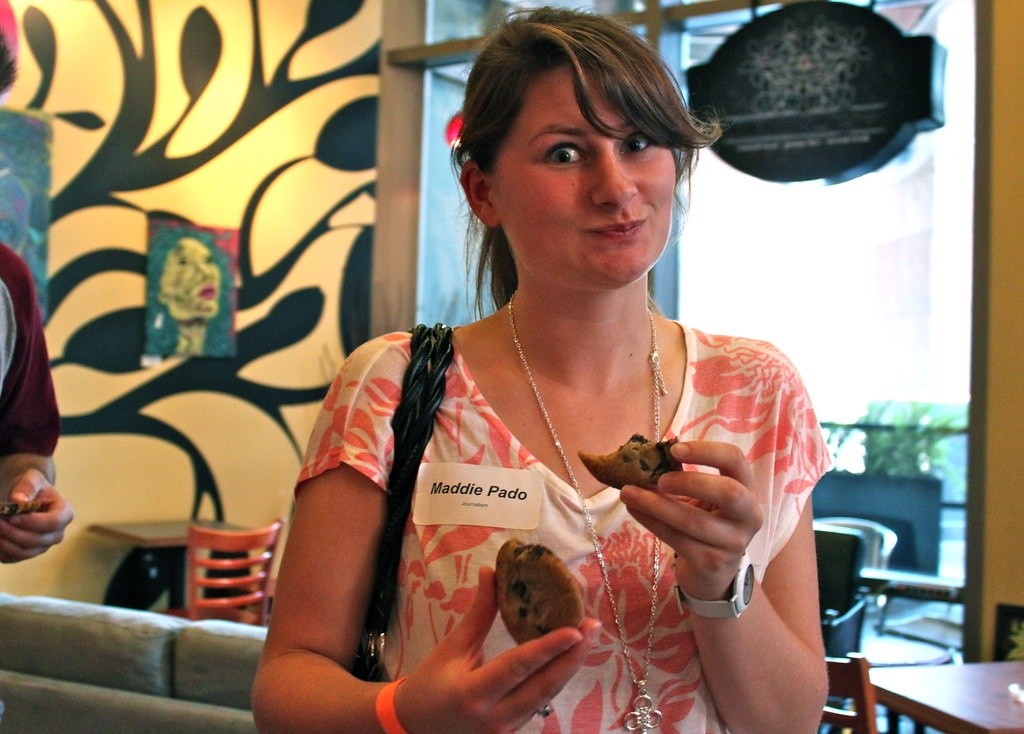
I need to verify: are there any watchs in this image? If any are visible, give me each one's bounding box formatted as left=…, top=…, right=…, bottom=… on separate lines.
left=671, top=548, right=755, bottom=620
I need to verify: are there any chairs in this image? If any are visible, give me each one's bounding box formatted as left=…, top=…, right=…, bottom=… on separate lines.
left=811, top=517, right=955, bottom=731
left=823, top=651, right=879, bottom=734
left=161, top=516, right=286, bottom=626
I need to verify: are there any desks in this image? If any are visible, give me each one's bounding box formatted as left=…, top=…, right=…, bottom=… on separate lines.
left=91, top=520, right=258, bottom=605
left=865, top=661, right=1024, bottom=733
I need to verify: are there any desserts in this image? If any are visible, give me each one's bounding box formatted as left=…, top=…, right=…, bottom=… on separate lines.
left=494, top=538, right=582, bottom=644
left=575, top=434, right=682, bottom=494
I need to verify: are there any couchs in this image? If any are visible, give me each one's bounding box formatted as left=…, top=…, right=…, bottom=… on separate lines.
left=0, top=593, right=269, bottom=734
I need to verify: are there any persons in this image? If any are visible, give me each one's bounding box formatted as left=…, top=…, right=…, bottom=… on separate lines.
left=0, top=242, right=74, bottom=563
left=250, top=5, right=833, bottom=734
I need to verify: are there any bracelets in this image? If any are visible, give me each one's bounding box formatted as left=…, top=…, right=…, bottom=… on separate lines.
left=375, top=674, right=412, bottom=734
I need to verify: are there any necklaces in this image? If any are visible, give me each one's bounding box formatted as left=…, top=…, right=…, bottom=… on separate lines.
left=505, top=292, right=669, bottom=734
